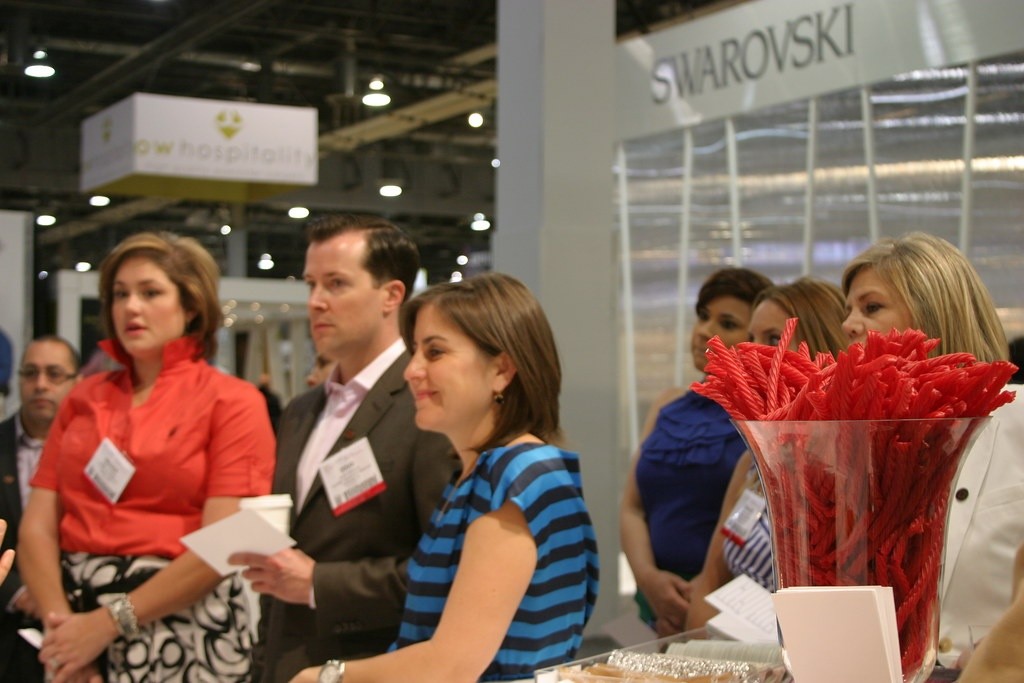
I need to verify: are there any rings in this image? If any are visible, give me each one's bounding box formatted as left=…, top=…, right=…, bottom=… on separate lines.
left=48, top=656, right=63, bottom=669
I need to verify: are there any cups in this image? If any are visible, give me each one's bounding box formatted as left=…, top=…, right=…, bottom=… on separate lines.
left=239, top=493, right=292, bottom=536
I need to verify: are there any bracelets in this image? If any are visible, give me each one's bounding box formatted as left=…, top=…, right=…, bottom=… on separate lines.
left=107, top=592, right=141, bottom=635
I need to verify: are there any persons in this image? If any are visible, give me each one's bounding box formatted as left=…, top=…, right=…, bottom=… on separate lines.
left=287, top=271, right=600, bottom=683
left=226, top=210, right=463, bottom=683
left=617, top=230, right=1024, bottom=683
left=2, top=223, right=280, bottom=683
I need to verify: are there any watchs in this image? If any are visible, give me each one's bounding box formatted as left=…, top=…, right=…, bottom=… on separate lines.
left=318, top=658, right=346, bottom=682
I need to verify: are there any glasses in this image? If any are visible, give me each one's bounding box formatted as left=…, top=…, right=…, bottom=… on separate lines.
left=18, top=367, right=76, bottom=385
